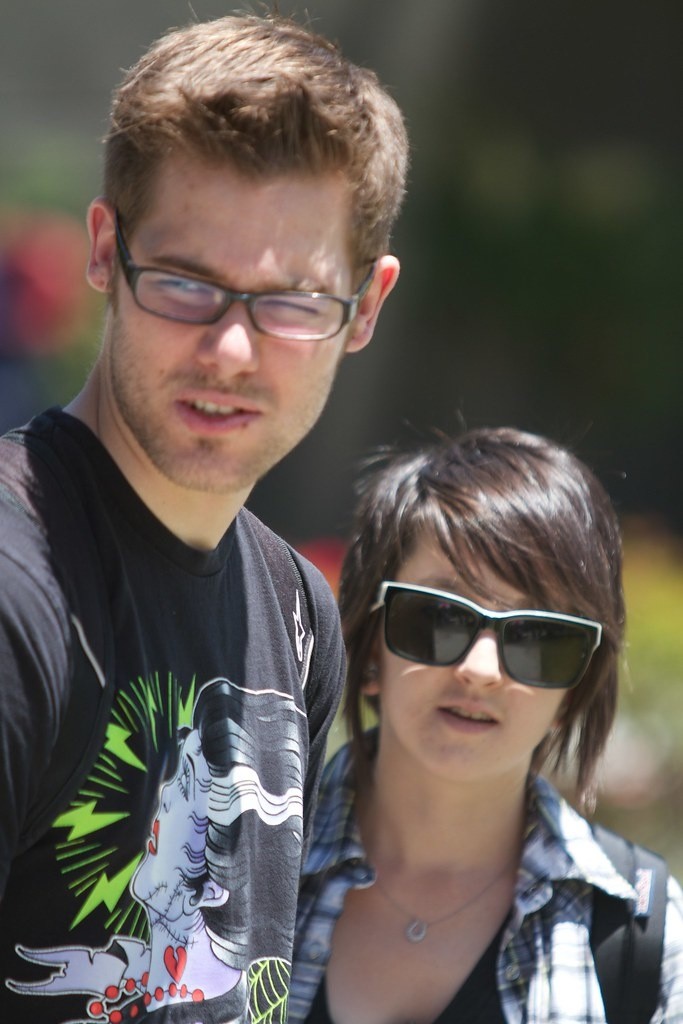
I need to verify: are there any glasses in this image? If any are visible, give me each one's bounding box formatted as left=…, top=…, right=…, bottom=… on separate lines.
left=114, top=209, right=376, bottom=341
left=369, top=581, right=603, bottom=690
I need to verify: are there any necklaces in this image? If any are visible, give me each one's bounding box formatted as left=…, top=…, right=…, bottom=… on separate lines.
left=377, top=869, right=509, bottom=942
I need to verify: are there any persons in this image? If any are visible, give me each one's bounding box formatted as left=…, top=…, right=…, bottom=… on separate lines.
left=0, top=5, right=411, bottom=1024
left=285, top=416, right=683, bottom=1023
